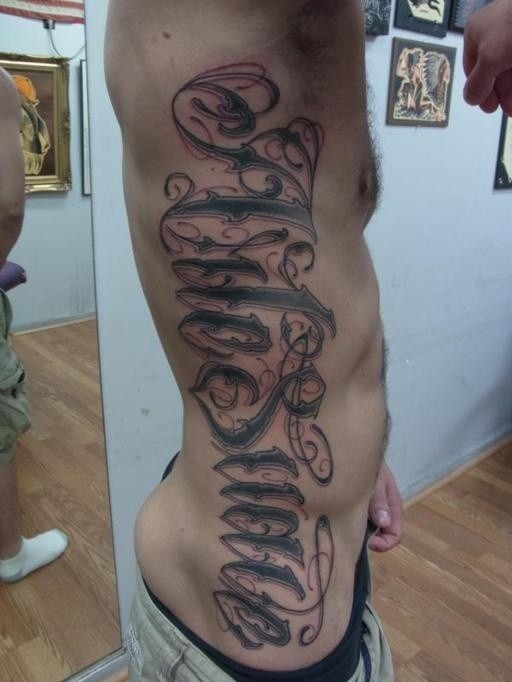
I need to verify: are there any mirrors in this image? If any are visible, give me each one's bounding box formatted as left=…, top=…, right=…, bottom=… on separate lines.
left=0, top=2, right=125, bottom=682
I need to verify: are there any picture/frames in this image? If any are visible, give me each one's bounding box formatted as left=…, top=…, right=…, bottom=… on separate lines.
left=80, top=59, right=91, bottom=196
left=395, top=0, right=451, bottom=38
left=493, top=112, right=512, bottom=190
left=0, top=54, right=73, bottom=193
left=386, top=37, right=457, bottom=127
left=449, top=0, right=493, bottom=32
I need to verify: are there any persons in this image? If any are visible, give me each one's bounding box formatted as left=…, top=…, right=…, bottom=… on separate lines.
left=103, top=1, right=512, bottom=682
left=0, top=65, right=69, bottom=581
